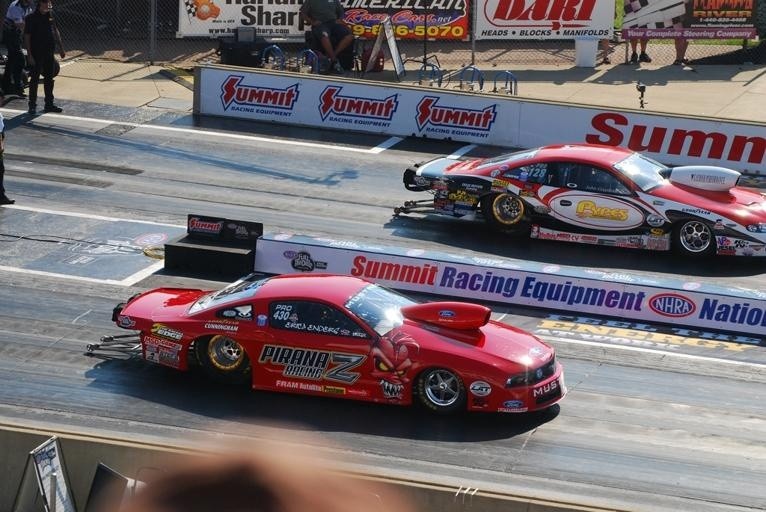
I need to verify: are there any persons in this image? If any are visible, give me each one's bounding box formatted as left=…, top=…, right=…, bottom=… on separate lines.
left=673, top=38, right=689, bottom=65
left=299, top=0, right=354, bottom=75
left=630, top=38, right=652, bottom=65
left=602, top=40, right=611, bottom=64
left=0, top=0, right=66, bottom=204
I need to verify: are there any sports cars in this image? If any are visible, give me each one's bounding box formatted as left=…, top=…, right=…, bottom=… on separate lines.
left=387, top=144, right=766, bottom=261
left=83, top=274, right=571, bottom=417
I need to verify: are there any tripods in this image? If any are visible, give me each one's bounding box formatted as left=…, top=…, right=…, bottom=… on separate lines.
left=402, top=0, right=441, bottom=76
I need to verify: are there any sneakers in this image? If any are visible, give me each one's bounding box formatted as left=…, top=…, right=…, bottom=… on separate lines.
left=640, top=54, right=651, bottom=62
left=631, top=53, right=638, bottom=62
